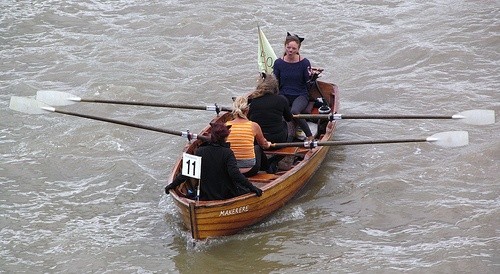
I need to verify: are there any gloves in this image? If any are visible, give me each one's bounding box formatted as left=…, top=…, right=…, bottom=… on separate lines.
left=249, top=185, right=263, bottom=197
left=164, top=181, right=176, bottom=194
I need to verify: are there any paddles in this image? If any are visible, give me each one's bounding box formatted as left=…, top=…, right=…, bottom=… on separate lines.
left=37, top=90, right=233, bottom=112
left=270, top=130, right=469, bottom=149
left=9, top=95, right=211, bottom=141
left=292, top=108, right=495, bottom=125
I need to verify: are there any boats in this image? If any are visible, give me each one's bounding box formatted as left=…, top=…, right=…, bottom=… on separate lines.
left=166, top=79, right=341, bottom=241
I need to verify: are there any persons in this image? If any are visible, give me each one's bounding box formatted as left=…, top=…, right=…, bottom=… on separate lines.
left=165, top=123, right=263, bottom=199
left=224, top=96, right=276, bottom=175
left=259, top=35, right=320, bottom=142
left=248, top=73, right=296, bottom=151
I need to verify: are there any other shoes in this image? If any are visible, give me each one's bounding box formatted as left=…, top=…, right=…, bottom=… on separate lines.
left=272, top=164, right=279, bottom=172
left=305, top=136, right=314, bottom=142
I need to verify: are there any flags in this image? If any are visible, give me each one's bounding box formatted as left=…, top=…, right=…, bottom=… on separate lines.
left=258, top=30, right=277, bottom=75
